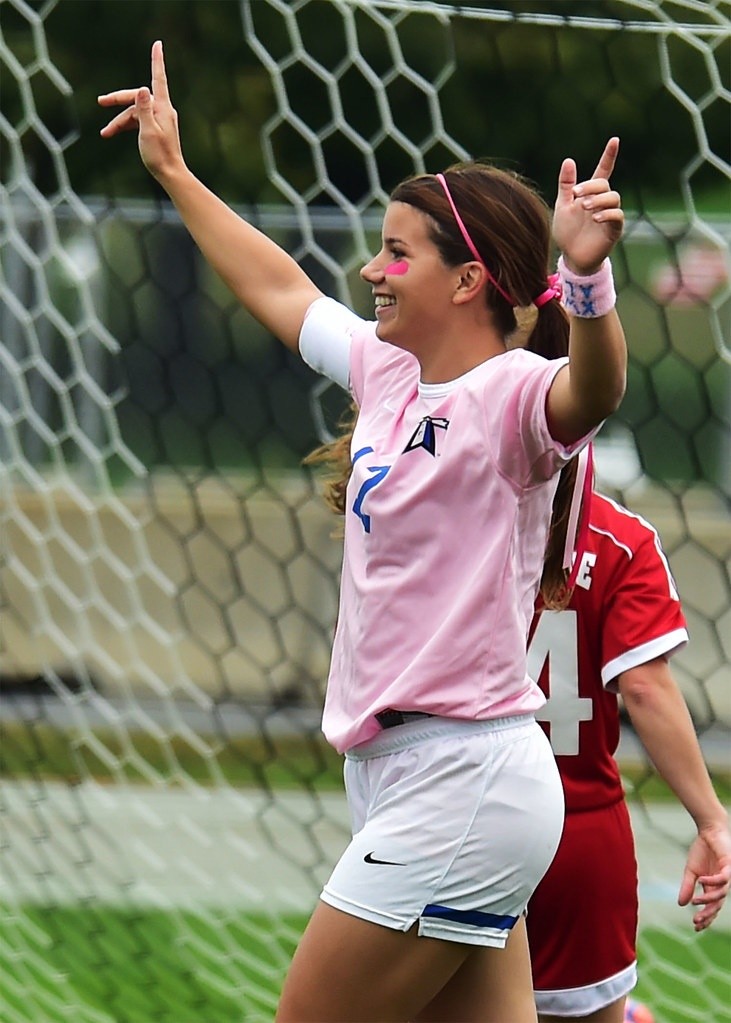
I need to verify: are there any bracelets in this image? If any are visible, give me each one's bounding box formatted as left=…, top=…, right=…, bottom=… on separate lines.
left=557, top=252, right=617, bottom=317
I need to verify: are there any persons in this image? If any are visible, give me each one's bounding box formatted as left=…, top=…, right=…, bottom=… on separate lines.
left=97, top=41, right=630, bottom=1023
left=301, top=303, right=731, bottom=1023
